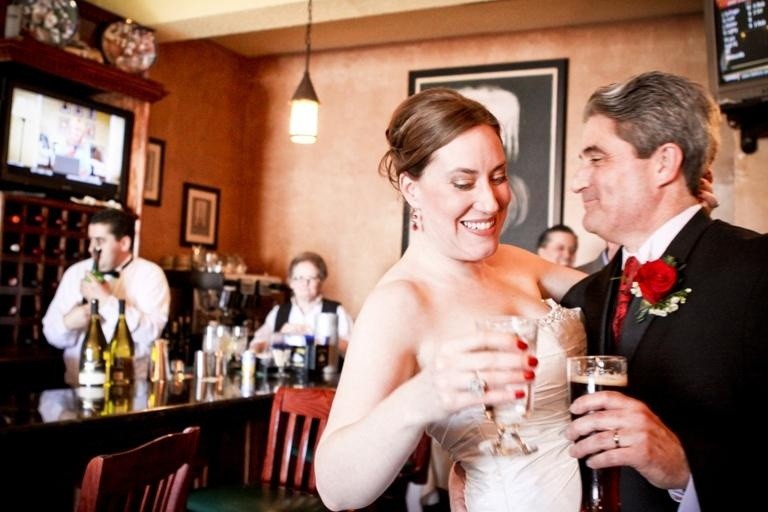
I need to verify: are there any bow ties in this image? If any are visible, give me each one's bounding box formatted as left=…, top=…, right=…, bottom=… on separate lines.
left=100, top=270, right=119, bottom=279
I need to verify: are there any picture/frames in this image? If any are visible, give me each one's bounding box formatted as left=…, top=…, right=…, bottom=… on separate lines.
left=143, top=136, right=167, bottom=206
left=180, top=181, right=221, bottom=249
left=400, top=58, right=568, bottom=260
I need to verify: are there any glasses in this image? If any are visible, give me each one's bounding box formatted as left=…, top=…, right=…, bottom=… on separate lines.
left=289, top=275, right=321, bottom=283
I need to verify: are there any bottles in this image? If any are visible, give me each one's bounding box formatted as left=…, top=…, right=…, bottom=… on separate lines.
left=196, top=378, right=230, bottom=402
left=241, top=351, right=255, bottom=377
left=192, top=322, right=232, bottom=378
left=109, top=387, right=132, bottom=416
left=148, top=382, right=165, bottom=407
left=241, top=377, right=254, bottom=398
left=79, top=387, right=107, bottom=419
left=79, top=301, right=108, bottom=387
left=110, top=299, right=135, bottom=386
left=149, top=339, right=170, bottom=381
left=170, top=317, right=191, bottom=361
left=314, top=313, right=338, bottom=373
left=82, top=248, right=102, bottom=304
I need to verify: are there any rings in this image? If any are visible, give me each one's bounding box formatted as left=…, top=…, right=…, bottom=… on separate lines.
left=614, top=427, right=621, bottom=450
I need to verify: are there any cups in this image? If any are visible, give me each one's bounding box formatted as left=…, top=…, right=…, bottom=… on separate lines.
left=257, top=347, right=272, bottom=378
left=474, top=313, right=540, bottom=458
left=565, top=355, right=630, bottom=512
left=194, top=351, right=208, bottom=379
left=241, top=352, right=257, bottom=398
left=273, top=349, right=291, bottom=379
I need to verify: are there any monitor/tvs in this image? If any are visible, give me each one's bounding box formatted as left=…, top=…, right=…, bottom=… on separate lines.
left=704, top=0, right=768, bottom=106
left=0, top=79, right=138, bottom=204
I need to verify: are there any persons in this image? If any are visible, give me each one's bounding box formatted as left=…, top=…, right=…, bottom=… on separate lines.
left=41, top=209, right=171, bottom=386
left=314, top=86, right=721, bottom=512
left=449, top=70, right=767, bottom=511
left=534, top=224, right=613, bottom=270
left=248, top=253, right=352, bottom=379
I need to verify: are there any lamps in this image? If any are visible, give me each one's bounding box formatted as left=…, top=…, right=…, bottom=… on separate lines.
left=287, top=69, right=321, bottom=146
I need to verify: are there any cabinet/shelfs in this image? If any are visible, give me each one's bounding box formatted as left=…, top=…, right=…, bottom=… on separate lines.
left=0, top=192, right=133, bottom=368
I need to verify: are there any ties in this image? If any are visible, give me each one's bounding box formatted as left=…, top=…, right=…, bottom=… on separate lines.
left=610, top=257, right=639, bottom=353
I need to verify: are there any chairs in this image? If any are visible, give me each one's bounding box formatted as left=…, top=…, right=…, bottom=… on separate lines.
left=183, top=387, right=338, bottom=512
left=75, top=426, right=200, bottom=512
